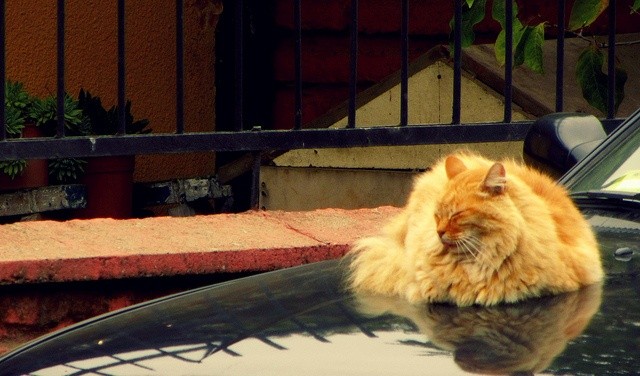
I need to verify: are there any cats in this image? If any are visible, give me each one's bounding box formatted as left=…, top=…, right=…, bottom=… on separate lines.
left=349, top=282, right=605, bottom=376
left=341, top=149, right=604, bottom=307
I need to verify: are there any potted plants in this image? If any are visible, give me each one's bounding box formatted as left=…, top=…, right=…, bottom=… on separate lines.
left=70, top=88, right=149, bottom=221
left=3, top=76, right=94, bottom=195
